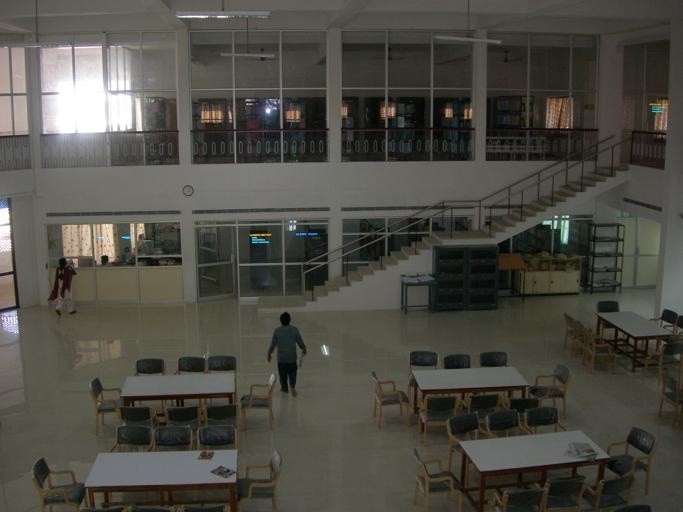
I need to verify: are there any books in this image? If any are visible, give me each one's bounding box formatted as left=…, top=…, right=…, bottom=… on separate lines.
left=568, top=440, right=598, bottom=457
left=209, top=465, right=235, bottom=478
left=197, top=451, right=213, bottom=460
left=562, top=448, right=595, bottom=462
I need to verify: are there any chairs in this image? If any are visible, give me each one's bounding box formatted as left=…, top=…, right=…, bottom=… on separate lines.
left=509, top=398, right=538, bottom=413
left=529, top=363, right=572, bottom=417
left=524, top=408, right=567, bottom=435
left=485, top=409, right=531, bottom=437
left=445, top=411, right=496, bottom=470
left=607, top=504, right=653, bottom=512
left=584, top=474, right=632, bottom=511
left=412, top=448, right=455, bottom=510
left=31, top=457, right=87, bottom=510
left=542, top=475, right=588, bottom=510
left=659, top=339, right=682, bottom=427
left=371, top=369, right=412, bottom=427
left=491, top=482, right=544, bottom=511
left=444, top=353, right=472, bottom=369
left=409, top=349, right=438, bottom=383
left=418, top=395, right=461, bottom=435
left=479, top=351, right=508, bottom=367
left=560, top=299, right=683, bottom=374
left=462, top=392, right=500, bottom=421
left=601, top=426, right=656, bottom=497
left=238, top=449, right=281, bottom=511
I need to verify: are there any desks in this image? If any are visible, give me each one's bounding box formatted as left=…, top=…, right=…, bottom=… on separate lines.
left=84, top=450, right=238, bottom=512
left=412, top=367, right=529, bottom=403
left=399, top=273, right=438, bottom=315
left=459, top=430, right=609, bottom=512
left=497, top=252, right=528, bottom=305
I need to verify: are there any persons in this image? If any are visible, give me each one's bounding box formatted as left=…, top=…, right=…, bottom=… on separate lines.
left=137, top=234, right=147, bottom=255
left=47, top=258, right=77, bottom=316
left=122, top=246, right=131, bottom=262
left=343, top=110, right=356, bottom=153
left=100, top=255, right=108, bottom=265
left=266, top=311, right=307, bottom=396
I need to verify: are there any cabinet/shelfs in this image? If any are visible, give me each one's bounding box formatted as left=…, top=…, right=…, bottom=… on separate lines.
left=431, top=243, right=503, bottom=314
left=513, top=252, right=586, bottom=296
left=142, top=95, right=534, bottom=162
left=580, top=220, right=627, bottom=297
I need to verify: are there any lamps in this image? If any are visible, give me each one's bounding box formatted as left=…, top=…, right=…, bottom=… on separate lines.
left=174, top=0, right=272, bottom=22
left=219, top=19, right=276, bottom=60
left=432, top=0, right=504, bottom=47
